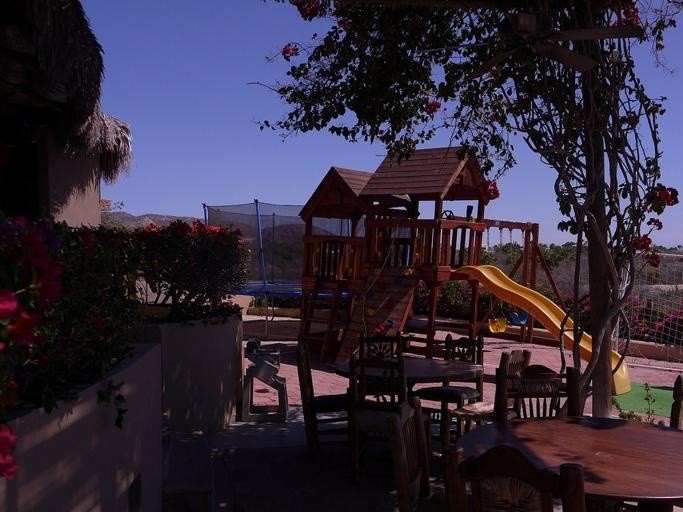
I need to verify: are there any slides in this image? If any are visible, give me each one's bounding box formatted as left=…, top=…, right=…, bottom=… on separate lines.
left=457, top=263, right=633, bottom=394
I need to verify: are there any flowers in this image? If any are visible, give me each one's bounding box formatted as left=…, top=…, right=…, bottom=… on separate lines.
left=0, top=220, right=249, bottom=483
